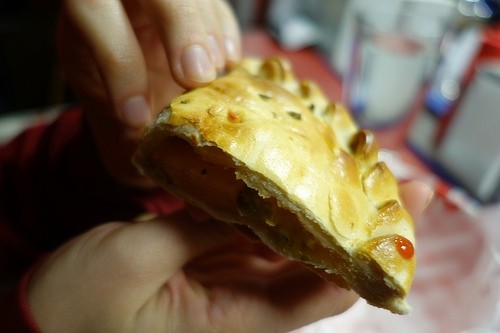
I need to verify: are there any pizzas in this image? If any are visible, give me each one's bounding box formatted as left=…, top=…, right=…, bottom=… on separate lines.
left=137, top=54, right=418, bottom=314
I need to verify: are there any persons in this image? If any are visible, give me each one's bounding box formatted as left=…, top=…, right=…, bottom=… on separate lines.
left=1, top=0, right=437, bottom=332
